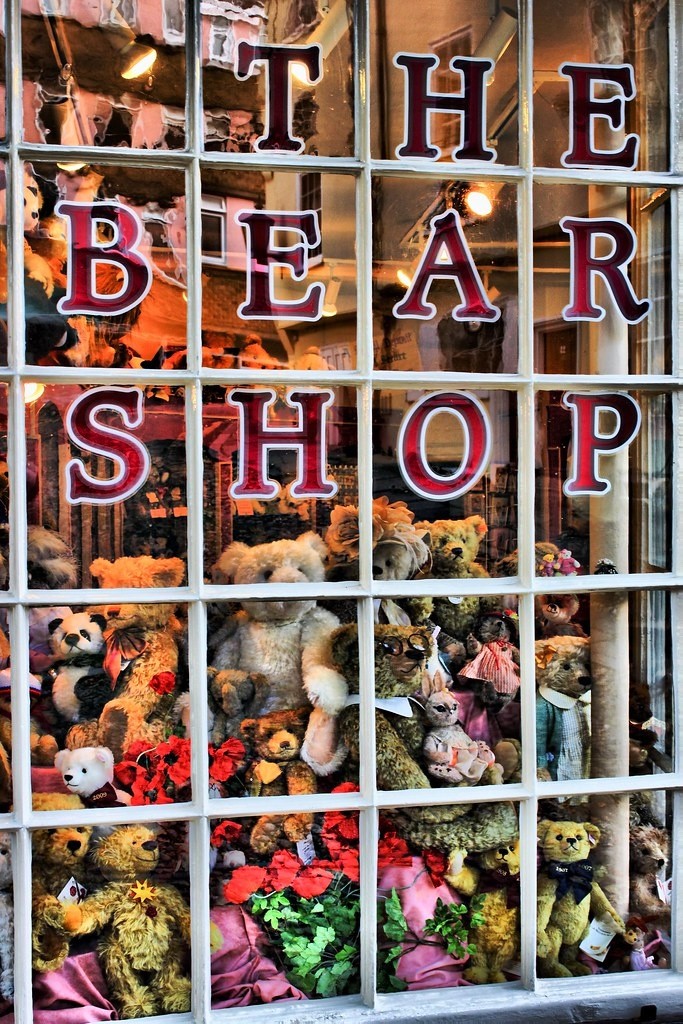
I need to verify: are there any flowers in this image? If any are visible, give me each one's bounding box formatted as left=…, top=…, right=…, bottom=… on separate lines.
left=210, top=780, right=489, bottom=997
left=128, top=878, right=157, bottom=904
left=594, top=557, right=618, bottom=574
left=326, top=496, right=415, bottom=560
left=113, top=671, right=246, bottom=806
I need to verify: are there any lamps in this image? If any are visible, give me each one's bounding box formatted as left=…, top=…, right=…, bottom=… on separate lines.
left=471, top=6, right=519, bottom=88
left=465, top=182, right=506, bottom=217
left=83, top=0, right=156, bottom=79
left=290, top=0, right=355, bottom=86
